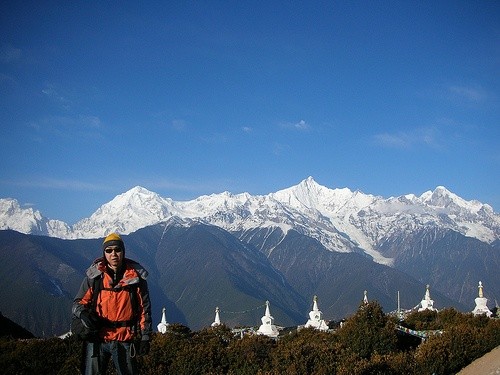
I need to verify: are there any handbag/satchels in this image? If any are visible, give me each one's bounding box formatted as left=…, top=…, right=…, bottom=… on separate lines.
left=70, top=313, right=99, bottom=342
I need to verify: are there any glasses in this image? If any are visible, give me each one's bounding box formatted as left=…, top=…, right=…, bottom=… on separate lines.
left=104, top=247, right=121, bottom=253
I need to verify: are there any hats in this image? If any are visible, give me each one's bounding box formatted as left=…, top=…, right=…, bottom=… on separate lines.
left=103, top=232, right=125, bottom=257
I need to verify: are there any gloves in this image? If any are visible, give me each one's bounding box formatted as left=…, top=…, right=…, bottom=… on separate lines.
left=139, top=341, right=150, bottom=357
left=80, top=311, right=97, bottom=329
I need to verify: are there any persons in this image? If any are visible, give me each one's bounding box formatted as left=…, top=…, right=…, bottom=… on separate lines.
left=72, top=233, right=153, bottom=375
